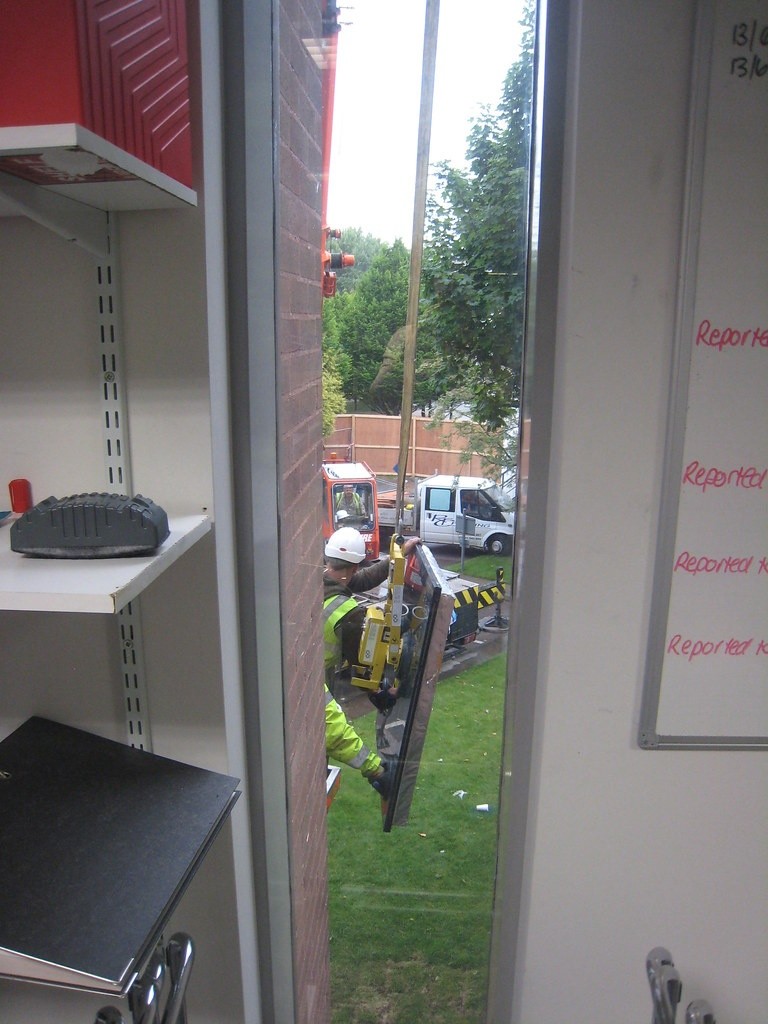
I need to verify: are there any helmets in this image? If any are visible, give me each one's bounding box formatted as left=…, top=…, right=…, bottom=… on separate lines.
left=324, top=526, right=367, bottom=564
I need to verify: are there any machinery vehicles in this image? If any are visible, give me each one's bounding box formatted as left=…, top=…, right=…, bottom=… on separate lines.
left=321, top=0, right=507, bottom=692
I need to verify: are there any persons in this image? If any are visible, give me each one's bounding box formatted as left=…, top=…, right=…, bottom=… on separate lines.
left=321, top=527, right=422, bottom=710
left=324, top=685, right=385, bottom=784
left=336, top=485, right=366, bottom=518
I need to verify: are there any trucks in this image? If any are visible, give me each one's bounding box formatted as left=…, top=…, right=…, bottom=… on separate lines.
left=375, top=475, right=514, bottom=558
